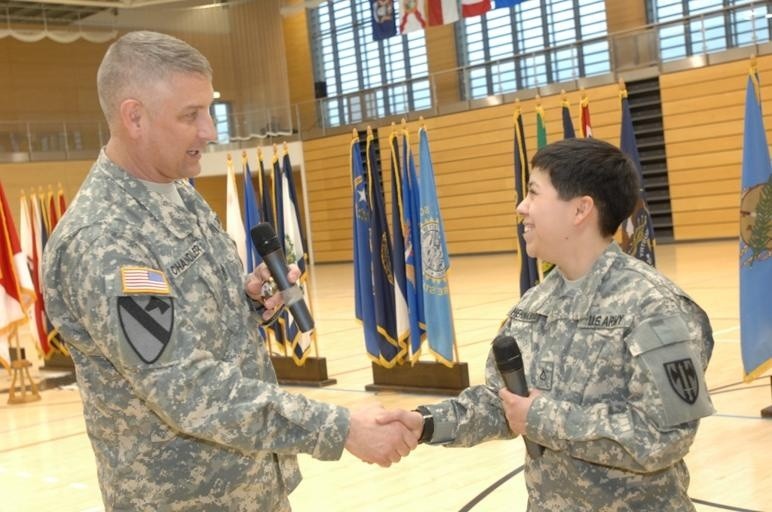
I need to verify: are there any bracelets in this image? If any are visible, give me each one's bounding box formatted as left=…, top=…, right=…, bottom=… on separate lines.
left=411, top=406, right=434, bottom=446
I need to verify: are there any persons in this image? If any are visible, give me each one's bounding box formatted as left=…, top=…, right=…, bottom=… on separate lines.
left=374, top=136, right=716, bottom=511
left=42, top=29, right=418, bottom=511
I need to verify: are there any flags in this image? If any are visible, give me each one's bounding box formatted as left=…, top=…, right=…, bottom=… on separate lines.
left=398, top=1, right=425, bottom=36
left=225, top=142, right=322, bottom=371
left=350, top=114, right=459, bottom=373
left=461, top=1, right=491, bottom=18
left=560, top=89, right=577, bottom=140
left=618, top=79, right=656, bottom=269
left=427, top=0, right=460, bottom=28
left=511, top=98, right=539, bottom=297
left=370, top=1, right=397, bottom=41
left=0, top=180, right=73, bottom=374
left=494, top=0, right=529, bottom=9
left=738, top=54, right=772, bottom=384
left=536, top=94, right=546, bottom=151
left=579, top=86, right=593, bottom=138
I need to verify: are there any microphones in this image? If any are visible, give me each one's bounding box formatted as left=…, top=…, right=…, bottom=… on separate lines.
left=250, top=222, right=314, bottom=334
left=492, top=335, right=543, bottom=459
